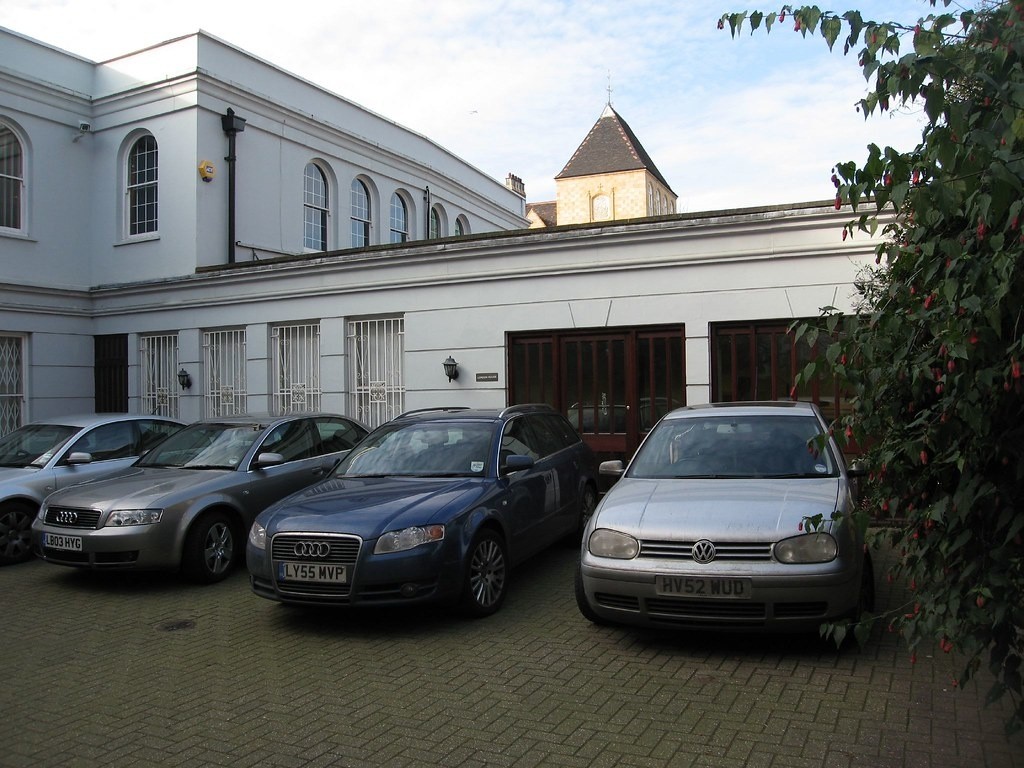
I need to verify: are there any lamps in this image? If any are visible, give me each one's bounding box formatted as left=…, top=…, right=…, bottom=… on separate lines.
left=176, top=368, right=192, bottom=391
left=442, top=355, right=459, bottom=383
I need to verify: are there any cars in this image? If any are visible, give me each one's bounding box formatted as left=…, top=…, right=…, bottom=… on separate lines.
left=573, top=398, right=873, bottom=637
left=27, top=410, right=389, bottom=588
left=0, top=412, right=219, bottom=570
left=245, top=402, right=601, bottom=620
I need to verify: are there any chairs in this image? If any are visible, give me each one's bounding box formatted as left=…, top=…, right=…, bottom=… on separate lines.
left=331, top=428, right=355, bottom=449
left=413, top=428, right=454, bottom=471
left=69, top=437, right=92, bottom=456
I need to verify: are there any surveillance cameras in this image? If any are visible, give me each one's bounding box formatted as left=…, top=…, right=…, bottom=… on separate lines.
left=78, top=120, right=90, bottom=131
